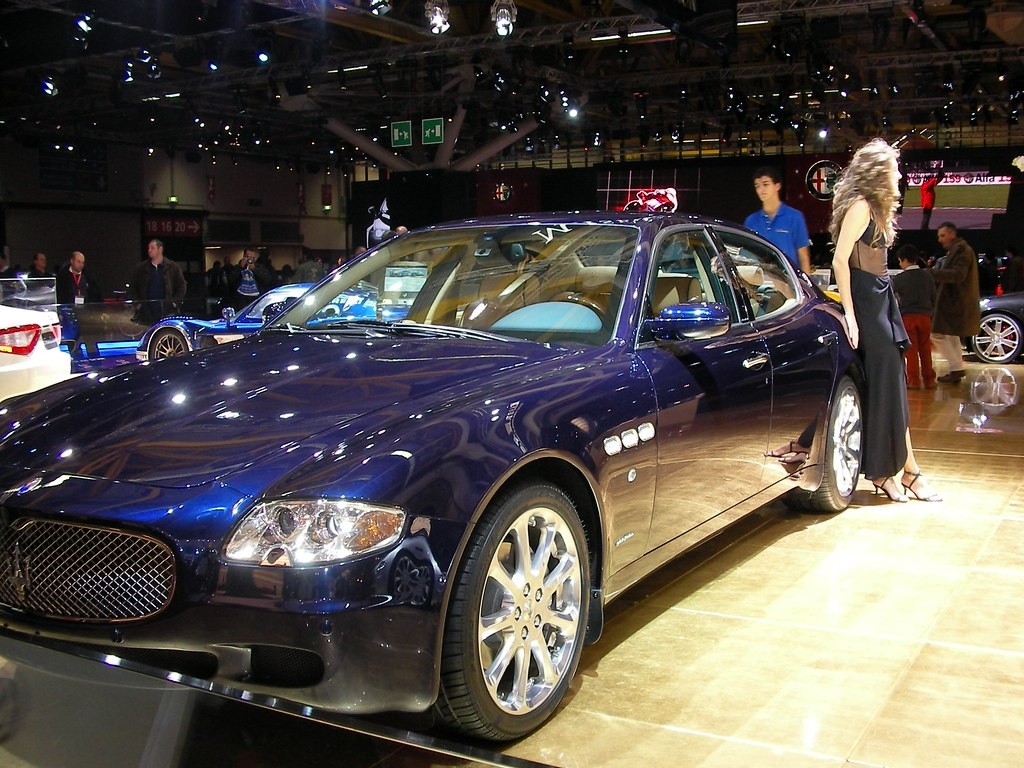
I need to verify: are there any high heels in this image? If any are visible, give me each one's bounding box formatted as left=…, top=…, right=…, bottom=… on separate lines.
left=901, top=470, right=943, bottom=502
left=872, top=476, right=911, bottom=502
left=763, top=439, right=810, bottom=459
left=778, top=453, right=807, bottom=465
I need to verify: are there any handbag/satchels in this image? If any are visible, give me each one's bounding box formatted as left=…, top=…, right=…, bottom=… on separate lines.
left=237, top=264, right=260, bottom=297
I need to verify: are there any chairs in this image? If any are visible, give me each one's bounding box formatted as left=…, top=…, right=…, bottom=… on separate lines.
left=646, top=272, right=705, bottom=324
left=457, top=269, right=542, bottom=332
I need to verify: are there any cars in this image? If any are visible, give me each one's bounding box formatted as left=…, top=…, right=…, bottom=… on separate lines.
left=0, top=270, right=427, bottom=404
left=966, top=290, right=1024, bottom=365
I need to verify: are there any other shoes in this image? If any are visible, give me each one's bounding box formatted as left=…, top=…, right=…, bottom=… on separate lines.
left=936, top=371, right=961, bottom=383
left=960, top=370, right=967, bottom=380
left=907, top=383, right=920, bottom=389
left=923, top=378, right=937, bottom=390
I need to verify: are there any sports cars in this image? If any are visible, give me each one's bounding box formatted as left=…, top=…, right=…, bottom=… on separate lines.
left=0, top=175, right=870, bottom=742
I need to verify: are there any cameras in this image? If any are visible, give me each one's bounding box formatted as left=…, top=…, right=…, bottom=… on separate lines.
left=246, top=257, right=254, bottom=264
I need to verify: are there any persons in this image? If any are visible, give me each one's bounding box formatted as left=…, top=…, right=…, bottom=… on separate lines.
left=743, top=170, right=810, bottom=279
left=919, top=173, right=945, bottom=229
left=933, top=222, right=981, bottom=383
left=767, top=410, right=817, bottom=463
left=1001, top=245, right=1024, bottom=293
left=891, top=245, right=938, bottom=389
left=832, top=137, right=942, bottom=504
left=0, top=240, right=367, bottom=326
left=397, top=225, right=407, bottom=233
left=710, top=253, right=853, bottom=349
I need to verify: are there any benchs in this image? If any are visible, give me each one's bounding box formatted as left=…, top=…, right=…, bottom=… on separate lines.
left=540, top=265, right=791, bottom=322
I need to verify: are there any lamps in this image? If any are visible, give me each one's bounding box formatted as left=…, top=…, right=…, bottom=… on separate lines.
left=1, top=1, right=1024, bottom=175
